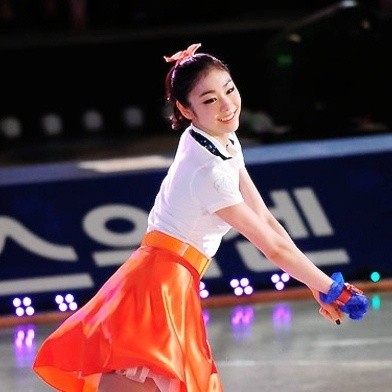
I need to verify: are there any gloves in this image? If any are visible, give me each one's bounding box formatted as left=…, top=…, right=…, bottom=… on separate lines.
left=319, top=271, right=369, bottom=320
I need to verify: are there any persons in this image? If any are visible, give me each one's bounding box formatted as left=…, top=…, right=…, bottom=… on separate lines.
left=31, top=44, right=368, bottom=392
